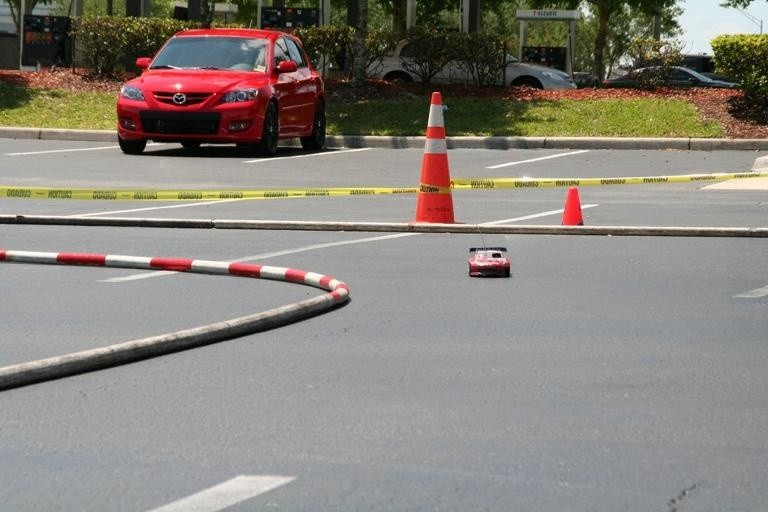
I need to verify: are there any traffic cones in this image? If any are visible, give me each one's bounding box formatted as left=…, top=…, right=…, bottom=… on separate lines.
left=403, top=90, right=464, bottom=225
left=561, top=187, right=585, bottom=226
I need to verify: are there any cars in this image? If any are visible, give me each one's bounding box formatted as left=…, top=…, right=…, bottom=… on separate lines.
left=113, top=28, right=329, bottom=159
left=364, top=34, right=578, bottom=91
left=574, top=61, right=742, bottom=90
